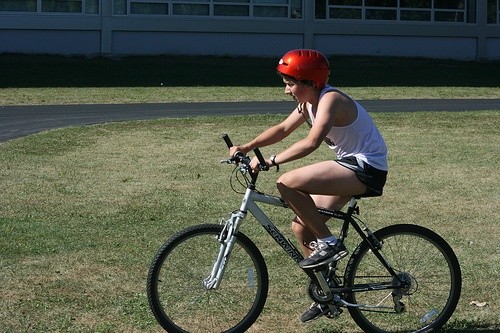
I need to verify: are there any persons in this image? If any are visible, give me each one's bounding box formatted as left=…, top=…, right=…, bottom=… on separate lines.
left=230, top=49, right=388, bottom=323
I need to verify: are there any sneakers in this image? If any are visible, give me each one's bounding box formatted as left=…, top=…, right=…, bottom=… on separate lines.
left=301, top=285, right=345, bottom=323
left=299, top=239, right=349, bottom=268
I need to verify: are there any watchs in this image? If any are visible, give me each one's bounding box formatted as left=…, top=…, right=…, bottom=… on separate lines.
left=270, top=154, right=280, bottom=173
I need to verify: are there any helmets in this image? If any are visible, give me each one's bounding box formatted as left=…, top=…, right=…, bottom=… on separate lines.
left=277, top=49, right=329, bottom=89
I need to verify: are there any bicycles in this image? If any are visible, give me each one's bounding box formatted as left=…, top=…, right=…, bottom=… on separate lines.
left=146, top=133, right=463, bottom=333
